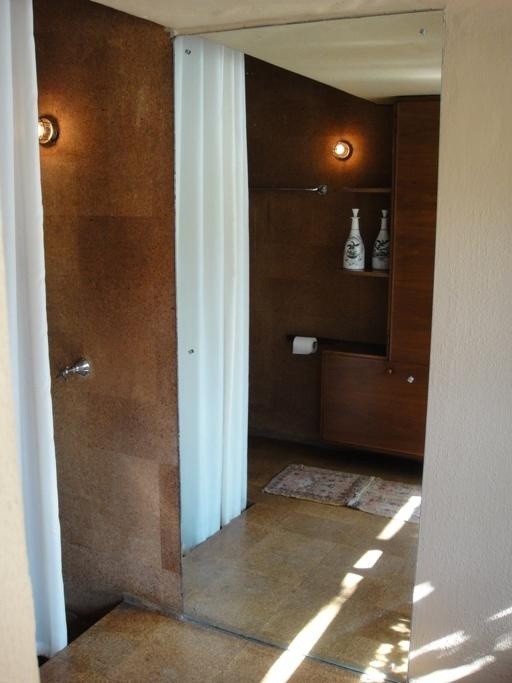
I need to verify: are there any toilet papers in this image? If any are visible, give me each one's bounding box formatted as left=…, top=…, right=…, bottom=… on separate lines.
left=292, top=336, right=318, bottom=355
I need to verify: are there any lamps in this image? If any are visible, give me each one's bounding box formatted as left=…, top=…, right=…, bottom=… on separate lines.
left=333, top=140, right=351, bottom=159
left=37, top=113, right=59, bottom=146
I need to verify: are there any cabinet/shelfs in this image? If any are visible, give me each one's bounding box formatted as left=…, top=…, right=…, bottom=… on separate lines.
left=319, top=94, right=440, bottom=456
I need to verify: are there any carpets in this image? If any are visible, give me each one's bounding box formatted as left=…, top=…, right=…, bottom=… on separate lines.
left=263, top=460, right=422, bottom=524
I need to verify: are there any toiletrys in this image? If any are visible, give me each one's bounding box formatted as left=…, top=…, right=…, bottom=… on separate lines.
left=372, top=209, right=391, bottom=270
left=342, top=208, right=365, bottom=271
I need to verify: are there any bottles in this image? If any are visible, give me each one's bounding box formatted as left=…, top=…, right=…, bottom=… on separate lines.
left=342, top=208, right=391, bottom=271
